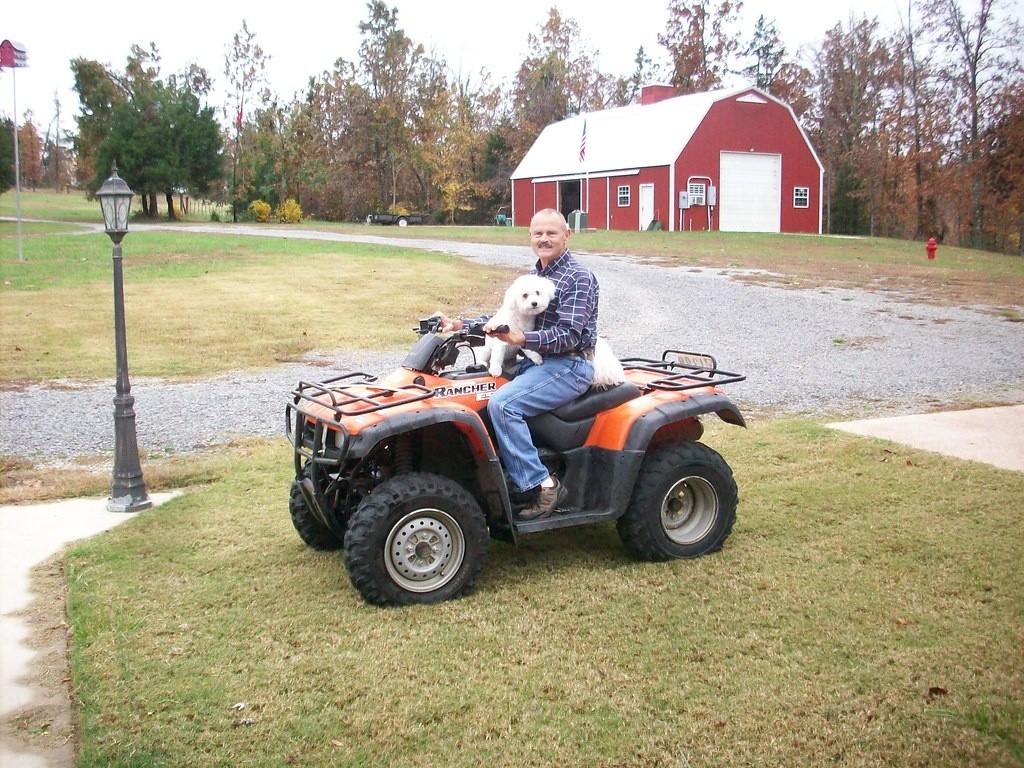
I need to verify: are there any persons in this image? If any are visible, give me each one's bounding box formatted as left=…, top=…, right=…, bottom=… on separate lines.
left=431, top=208, right=600, bottom=521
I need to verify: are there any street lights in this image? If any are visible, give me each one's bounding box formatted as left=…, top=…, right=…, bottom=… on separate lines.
left=92, top=157, right=155, bottom=514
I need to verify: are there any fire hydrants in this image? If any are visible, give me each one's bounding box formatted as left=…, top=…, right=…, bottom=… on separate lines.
left=925, top=237, right=939, bottom=261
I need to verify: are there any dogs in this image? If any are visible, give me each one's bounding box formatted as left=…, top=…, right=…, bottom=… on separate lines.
left=483, top=274, right=625, bottom=390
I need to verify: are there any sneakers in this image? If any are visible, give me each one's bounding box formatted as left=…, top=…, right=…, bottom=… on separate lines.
left=519, top=472, right=569, bottom=521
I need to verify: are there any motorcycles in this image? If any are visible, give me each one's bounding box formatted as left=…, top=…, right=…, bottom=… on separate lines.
left=281, top=317, right=748, bottom=616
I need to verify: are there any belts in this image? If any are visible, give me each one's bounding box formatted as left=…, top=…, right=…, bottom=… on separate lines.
left=564, top=350, right=594, bottom=361
